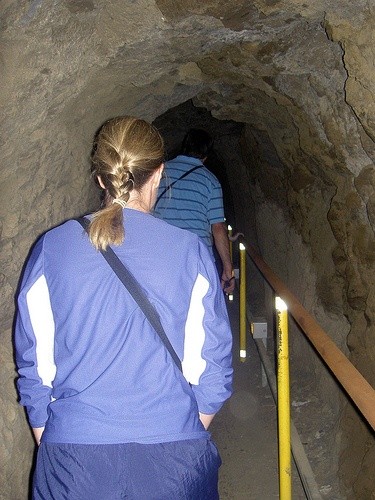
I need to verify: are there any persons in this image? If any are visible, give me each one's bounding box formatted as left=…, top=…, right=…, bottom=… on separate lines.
left=10, top=110, right=235, bottom=500
left=150, top=126, right=234, bottom=300
